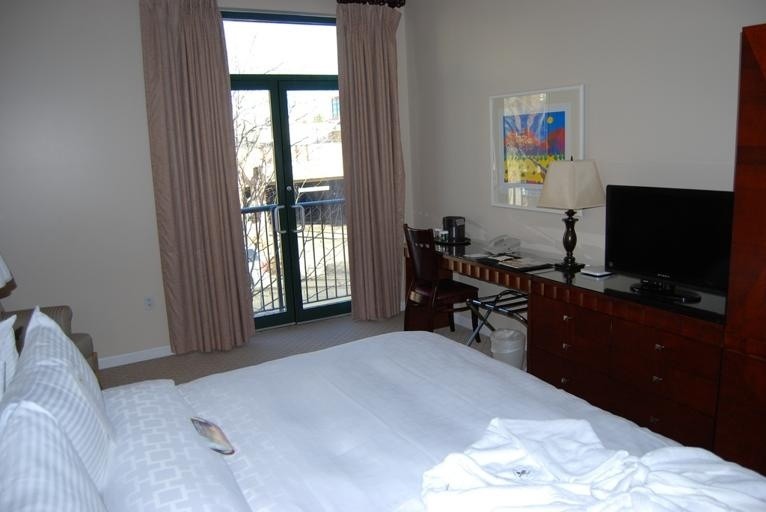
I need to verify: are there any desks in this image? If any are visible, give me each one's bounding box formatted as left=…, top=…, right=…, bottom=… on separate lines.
left=403, top=236, right=571, bottom=333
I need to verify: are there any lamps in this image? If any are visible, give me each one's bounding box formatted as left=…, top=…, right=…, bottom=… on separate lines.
left=534, top=157, right=608, bottom=275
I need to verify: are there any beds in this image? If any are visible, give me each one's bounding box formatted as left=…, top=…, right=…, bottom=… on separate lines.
left=0, top=311, right=765, bottom=511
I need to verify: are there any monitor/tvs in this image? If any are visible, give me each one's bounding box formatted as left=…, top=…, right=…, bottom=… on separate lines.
left=604, top=184, right=734, bottom=305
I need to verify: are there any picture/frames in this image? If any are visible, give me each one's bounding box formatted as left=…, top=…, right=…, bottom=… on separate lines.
left=488, top=81, right=587, bottom=218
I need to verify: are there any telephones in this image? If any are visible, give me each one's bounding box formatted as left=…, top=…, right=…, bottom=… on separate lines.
left=482, top=234, right=520, bottom=255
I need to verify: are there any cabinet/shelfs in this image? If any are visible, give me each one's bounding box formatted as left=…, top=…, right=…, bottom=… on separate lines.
left=523, top=264, right=726, bottom=454
left=726, top=22, right=766, bottom=482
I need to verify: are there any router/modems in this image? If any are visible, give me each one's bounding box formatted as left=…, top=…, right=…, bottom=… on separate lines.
left=580, top=264, right=612, bottom=278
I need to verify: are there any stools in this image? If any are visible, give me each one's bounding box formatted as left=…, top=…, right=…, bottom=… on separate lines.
left=465, top=290, right=529, bottom=347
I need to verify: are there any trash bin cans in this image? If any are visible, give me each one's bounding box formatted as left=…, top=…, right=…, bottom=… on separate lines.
left=490, top=329, right=525, bottom=370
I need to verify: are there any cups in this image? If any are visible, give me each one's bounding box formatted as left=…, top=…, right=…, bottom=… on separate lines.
left=434, top=229, right=440, bottom=240
left=439, top=230, right=449, bottom=242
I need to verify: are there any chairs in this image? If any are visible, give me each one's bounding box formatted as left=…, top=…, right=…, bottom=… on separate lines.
left=0, top=304, right=100, bottom=381
left=401, top=223, right=482, bottom=345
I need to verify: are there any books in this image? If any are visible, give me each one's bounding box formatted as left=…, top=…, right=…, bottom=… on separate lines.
left=581, top=266, right=614, bottom=278
left=498, top=257, right=546, bottom=269
left=191, top=418, right=235, bottom=455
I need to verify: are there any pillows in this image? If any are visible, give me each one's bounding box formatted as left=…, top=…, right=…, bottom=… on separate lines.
left=0, top=398, right=108, bottom=512
left=0, top=311, right=21, bottom=394
left=0, top=303, right=112, bottom=512
